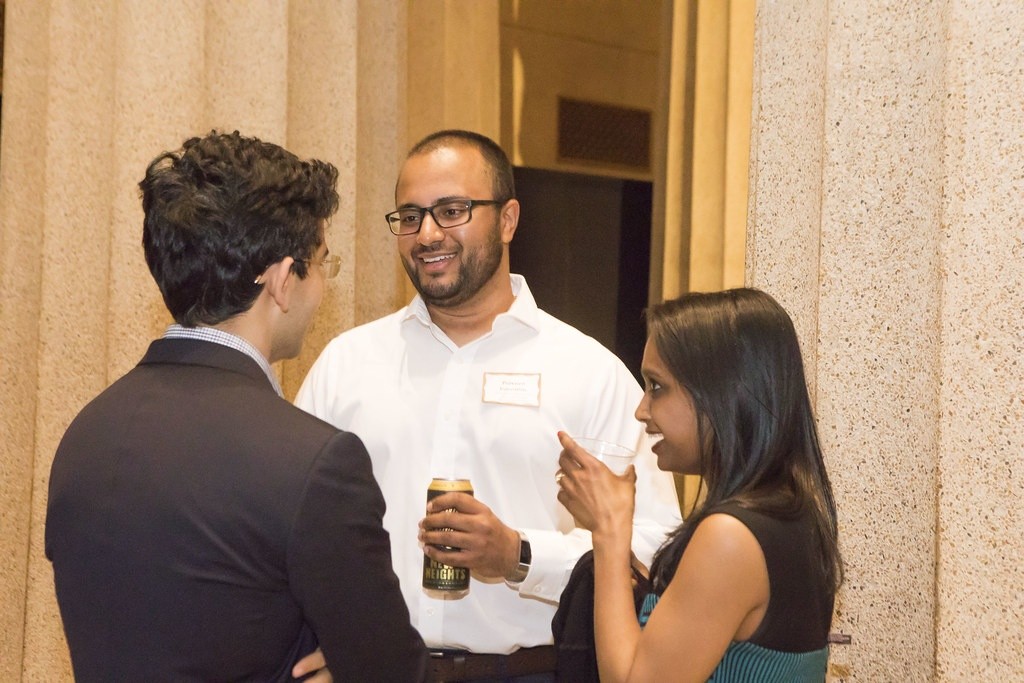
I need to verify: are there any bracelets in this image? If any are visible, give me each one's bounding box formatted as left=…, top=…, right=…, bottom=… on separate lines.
left=506, top=530, right=532, bottom=582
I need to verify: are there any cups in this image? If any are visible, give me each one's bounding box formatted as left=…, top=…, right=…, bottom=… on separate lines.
left=573, top=437, right=636, bottom=474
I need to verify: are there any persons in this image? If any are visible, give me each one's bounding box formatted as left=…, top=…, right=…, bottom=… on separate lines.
left=293, top=129, right=684, bottom=683
left=45, top=129, right=433, bottom=683
left=557, top=288, right=843, bottom=683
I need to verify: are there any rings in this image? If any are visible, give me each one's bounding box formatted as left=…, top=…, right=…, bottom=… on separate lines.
left=555, top=473, right=565, bottom=484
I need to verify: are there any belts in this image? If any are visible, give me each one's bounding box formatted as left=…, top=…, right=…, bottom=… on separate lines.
left=430, top=643, right=560, bottom=683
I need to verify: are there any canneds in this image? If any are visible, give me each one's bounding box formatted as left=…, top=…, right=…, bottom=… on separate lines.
left=421, top=477, right=473, bottom=600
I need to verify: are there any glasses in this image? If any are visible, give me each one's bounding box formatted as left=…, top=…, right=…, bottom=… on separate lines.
left=385, top=200, right=506, bottom=236
left=253, top=253, right=341, bottom=284
